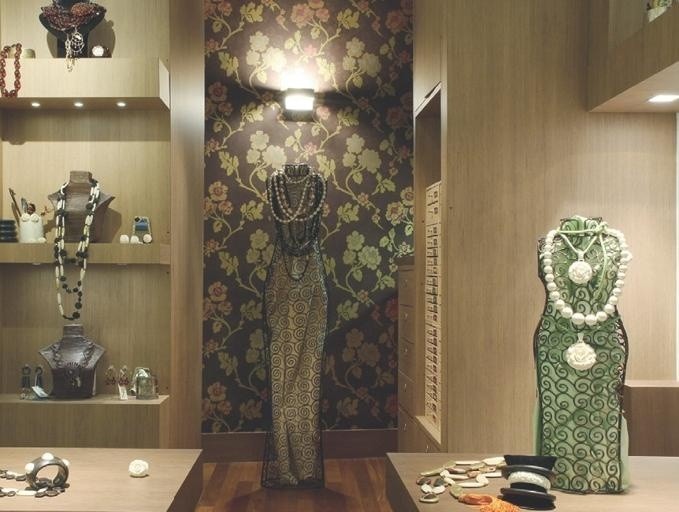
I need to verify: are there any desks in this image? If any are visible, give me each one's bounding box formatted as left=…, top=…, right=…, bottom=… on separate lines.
left=0, top=446, right=203, bottom=512
left=383, top=451, right=679, bottom=512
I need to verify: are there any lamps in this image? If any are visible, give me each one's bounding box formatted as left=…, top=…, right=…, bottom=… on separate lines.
left=283, top=89, right=315, bottom=112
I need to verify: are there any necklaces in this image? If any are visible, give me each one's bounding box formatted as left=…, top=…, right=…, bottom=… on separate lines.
left=268, top=169, right=328, bottom=223
left=0, top=42, right=22, bottom=98
left=52, top=336, right=96, bottom=391
left=38, top=3, right=101, bottom=66
left=54, top=179, right=100, bottom=319
left=541, top=226, right=630, bottom=371
left=561, top=231, right=600, bottom=286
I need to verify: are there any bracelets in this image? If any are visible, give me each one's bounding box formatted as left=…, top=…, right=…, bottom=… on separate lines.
left=499, top=464, right=556, bottom=502
left=24, top=452, right=69, bottom=489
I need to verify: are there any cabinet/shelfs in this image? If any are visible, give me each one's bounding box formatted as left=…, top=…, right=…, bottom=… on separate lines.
left=424, top=181, right=442, bottom=430
left=398, top=405, right=442, bottom=452
left=0, top=57, right=171, bottom=450
left=413, top=0, right=442, bottom=120
left=397, top=271, right=425, bottom=415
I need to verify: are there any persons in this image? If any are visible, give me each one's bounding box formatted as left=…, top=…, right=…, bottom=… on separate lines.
left=259, top=163, right=332, bottom=489
left=532, top=218, right=630, bottom=497
left=39, top=1, right=107, bottom=58
left=48, top=169, right=116, bottom=244
left=38, top=323, right=107, bottom=397
